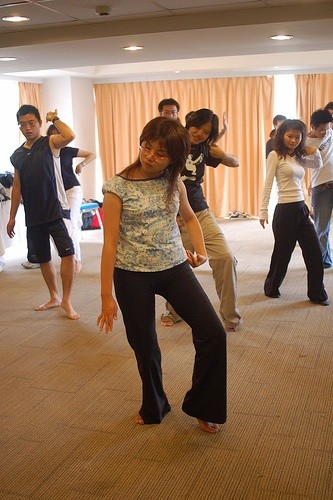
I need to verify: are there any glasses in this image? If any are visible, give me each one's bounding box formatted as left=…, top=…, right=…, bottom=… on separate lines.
left=161, top=110, right=179, bottom=116
left=139, top=143, right=170, bottom=164
left=316, top=128, right=332, bottom=134
left=19, top=120, right=37, bottom=127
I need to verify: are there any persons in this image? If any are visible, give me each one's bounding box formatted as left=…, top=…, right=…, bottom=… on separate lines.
left=95, top=116, right=227, bottom=432
left=18, top=125, right=96, bottom=274
left=148, top=98, right=332, bottom=331
left=5, top=105, right=81, bottom=319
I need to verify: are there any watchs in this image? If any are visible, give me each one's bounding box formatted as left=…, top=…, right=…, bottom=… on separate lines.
left=51, top=117, right=58, bottom=123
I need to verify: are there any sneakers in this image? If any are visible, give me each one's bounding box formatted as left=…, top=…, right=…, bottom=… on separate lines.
left=21, top=260, right=41, bottom=269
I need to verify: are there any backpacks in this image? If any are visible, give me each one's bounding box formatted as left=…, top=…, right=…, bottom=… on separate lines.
left=80, top=211, right=94, bottom=230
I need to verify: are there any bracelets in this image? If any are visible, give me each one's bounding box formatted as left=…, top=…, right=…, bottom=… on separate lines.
left=80, top=161, right=85, bottom=167
